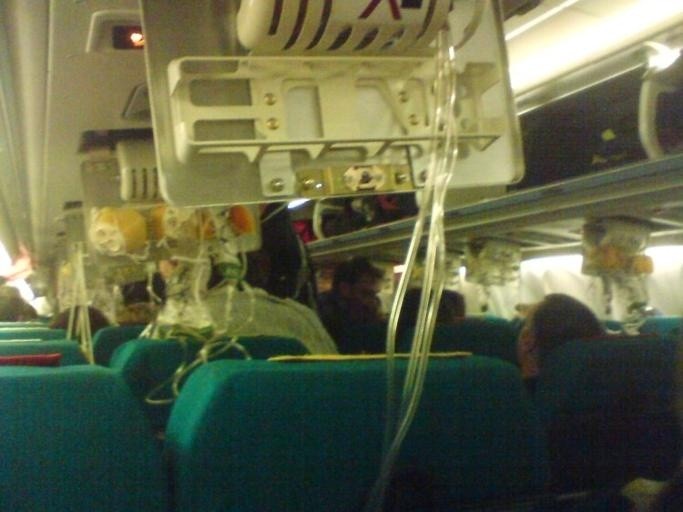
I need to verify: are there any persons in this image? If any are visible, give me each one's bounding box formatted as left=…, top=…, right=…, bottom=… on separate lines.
left=609, top=332, right=683, bottom=510
left=517, top=297, right=606, bottom=377
left=60, top=225, right=465, bottom=349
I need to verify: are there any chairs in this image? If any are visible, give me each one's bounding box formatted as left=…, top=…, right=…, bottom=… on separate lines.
left=464, top=307, right=681, bottom=509
left=155, top=351, right=527, bottom=509
left=1, top=308, right=150, bottom=366
left=107, top=336, right=313, bottom=435
left=2, top=366, right=172, bottom=510
